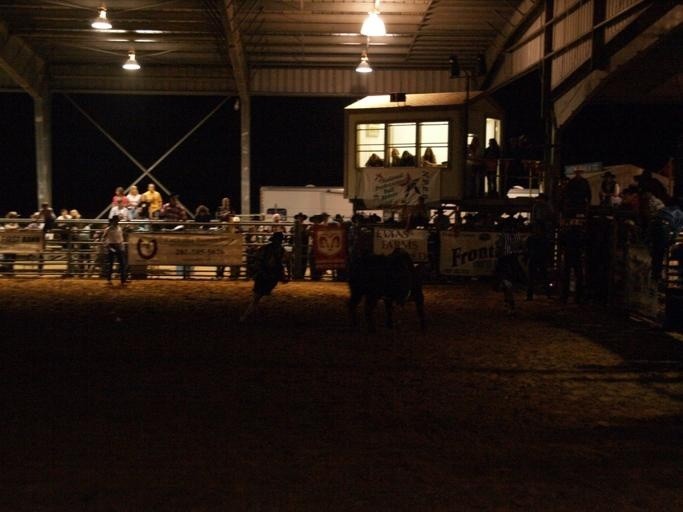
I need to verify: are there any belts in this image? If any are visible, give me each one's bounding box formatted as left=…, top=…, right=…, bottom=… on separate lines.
left=651, top=218, right=669, bottom=225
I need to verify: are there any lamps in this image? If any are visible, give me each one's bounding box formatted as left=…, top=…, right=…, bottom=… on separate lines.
left=356, top=48, right=372, bottom=73
left=361, top=5, right=386, bottom=36
left=92, top=6, right=112, bottom=29
left=123, top=51, right=140, bottom=71
left=235, top=99, right=240, bottom=109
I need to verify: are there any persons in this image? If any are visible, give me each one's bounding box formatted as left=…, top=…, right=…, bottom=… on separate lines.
left=1, top=184, right=353, bottom=287
left=240, top=232, right=288, bottom=322
left=351, top=136, right=681, bottom=329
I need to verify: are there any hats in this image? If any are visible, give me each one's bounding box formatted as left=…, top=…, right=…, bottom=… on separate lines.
left=634, top=170, right=651, bottom=181
left=435, top=210, right=444, bottom=214
left=601, top=171, right=616, bottom=179
left=110, top=216, right=120, bottom=222
left=269, top=232, right=284, bottom=241
left=573, top=166, right=585, bottom=173
left=292, top=212, right=345, bottom=223
left=369, top=214, right=381, bottom=222
left=352, top=214, right=363, bottom=223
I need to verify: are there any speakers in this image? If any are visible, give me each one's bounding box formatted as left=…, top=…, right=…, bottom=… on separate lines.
left=474, top=54, right=486, bottom=77
left=448, top=57, right=459, bottom=77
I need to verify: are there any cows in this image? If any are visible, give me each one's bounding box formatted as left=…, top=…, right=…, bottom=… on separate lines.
left=340, top=251, right=429, bottom=337
